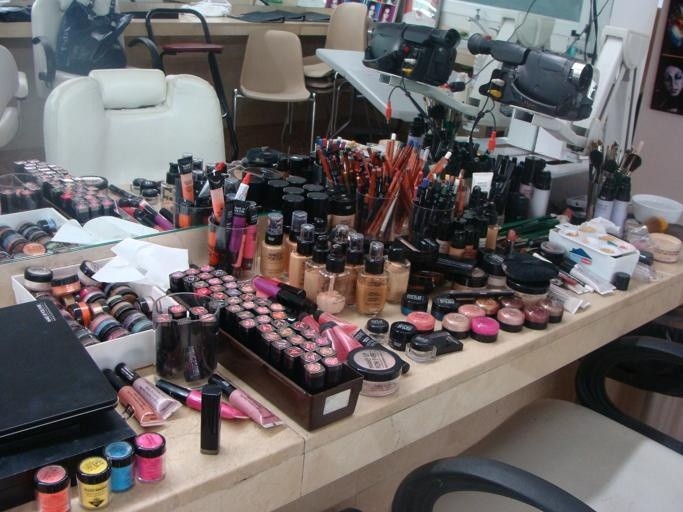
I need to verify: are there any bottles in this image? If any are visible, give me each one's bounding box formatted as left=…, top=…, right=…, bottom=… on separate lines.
left=34, top=432, right=165, bottom=511
left=587, top=165, right=630, bottom=227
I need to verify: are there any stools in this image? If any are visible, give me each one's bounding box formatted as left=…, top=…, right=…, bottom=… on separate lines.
left=146, top=8, right=240, bottom=159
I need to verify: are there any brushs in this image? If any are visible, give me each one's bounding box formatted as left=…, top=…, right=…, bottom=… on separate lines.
left=583, top=139, right=644, bottom=219
left=357, top=134, right=469, bottom=236
left=312, top=133, right=372, bottom=188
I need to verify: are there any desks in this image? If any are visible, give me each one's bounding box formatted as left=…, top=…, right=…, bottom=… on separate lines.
left=313, top=46, right=455, bottom=142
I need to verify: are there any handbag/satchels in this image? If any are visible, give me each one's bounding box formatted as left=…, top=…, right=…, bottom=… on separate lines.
left=55, top=0, right=133, bottom=74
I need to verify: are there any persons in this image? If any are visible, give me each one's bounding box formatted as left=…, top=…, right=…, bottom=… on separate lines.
left=650, top=62, right=683, bottom=114
left=381, top=6, right=391, bottom=22
left=367, top=2, right=376, bottom=22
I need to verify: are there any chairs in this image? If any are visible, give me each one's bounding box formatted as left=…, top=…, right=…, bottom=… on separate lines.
left=288, top=1, right=369, bottom=136
left=2, top=43, right=32, bottom=154
left=232, top=29, right=315, bottom=147
left=32, top=1, right=161, bottom=97
left=44, top=69, right=226, bottom=192
left=394, top=333, right=681, bottom=510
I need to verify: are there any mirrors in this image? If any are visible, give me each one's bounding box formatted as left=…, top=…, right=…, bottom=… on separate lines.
left=1, top=0, right=664, bottom=261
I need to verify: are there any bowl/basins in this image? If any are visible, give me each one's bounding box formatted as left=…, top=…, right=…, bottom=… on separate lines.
left=631, top=191, right=683, bottom=227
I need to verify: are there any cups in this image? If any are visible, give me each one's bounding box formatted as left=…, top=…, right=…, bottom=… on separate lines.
left=407, top=197, right=456, bottom=243
left=0, top=172, right=41, bottom=215
left=310, top=159, right=355, bottom=196
left=204, top=217, right=257, bottom=282
left=172, top=202, right=212, bottom=228
left=354, top=185, right=396, bottom=243
left=152, top=292, right=221, bottom=390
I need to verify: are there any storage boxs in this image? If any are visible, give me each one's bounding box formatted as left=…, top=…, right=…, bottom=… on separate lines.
left=8, top=253, right=191, bottom=377
left=0, top=205, right=84, bottom=261
left=163, top=278, right=366, bottom=431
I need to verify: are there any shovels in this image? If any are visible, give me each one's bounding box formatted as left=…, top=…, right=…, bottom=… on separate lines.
left=227, top=10, right=331, bottom=23
